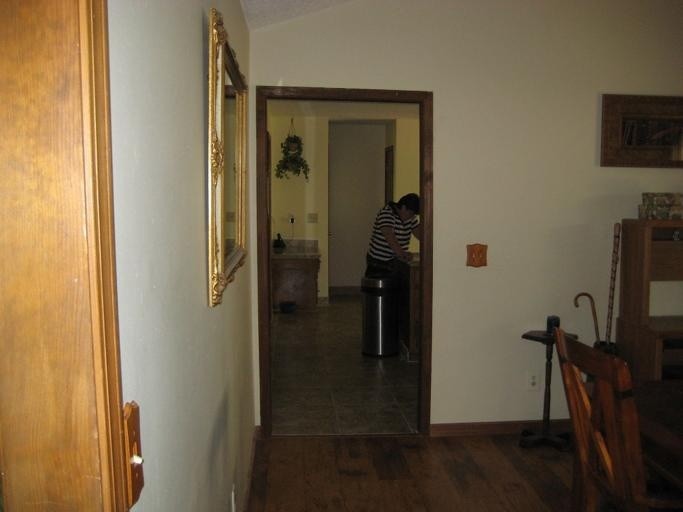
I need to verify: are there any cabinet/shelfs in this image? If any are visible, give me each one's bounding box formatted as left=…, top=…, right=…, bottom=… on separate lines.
left=613, top=220, right=683, bottom=388
left=270, top=258, right=321, bottom=310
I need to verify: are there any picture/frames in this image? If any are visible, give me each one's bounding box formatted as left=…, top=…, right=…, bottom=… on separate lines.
left=599, top=94, right=683, bottom=169
left=206, top=8, right=247, bottom=309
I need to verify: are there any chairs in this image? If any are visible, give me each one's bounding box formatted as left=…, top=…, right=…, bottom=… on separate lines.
left=553, top=324, right=683, bottom=512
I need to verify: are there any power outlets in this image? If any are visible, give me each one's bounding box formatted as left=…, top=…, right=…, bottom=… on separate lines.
left=527, top=375, right=540, bottom=391
left=231, top=485, right=237, bottom=512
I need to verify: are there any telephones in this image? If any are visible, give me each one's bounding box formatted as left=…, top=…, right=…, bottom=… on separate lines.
left=274, top=233, right=285, bottom=248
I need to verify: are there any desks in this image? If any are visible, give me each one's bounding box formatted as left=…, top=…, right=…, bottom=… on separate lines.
left=635, top=379, right=683, bottom=458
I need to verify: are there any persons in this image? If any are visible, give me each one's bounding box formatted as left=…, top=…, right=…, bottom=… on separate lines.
left=365, top=192, right=419, bottom=272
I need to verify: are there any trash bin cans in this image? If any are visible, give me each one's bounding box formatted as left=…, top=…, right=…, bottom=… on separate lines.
left=361, top=278, right=398, bottom=359
